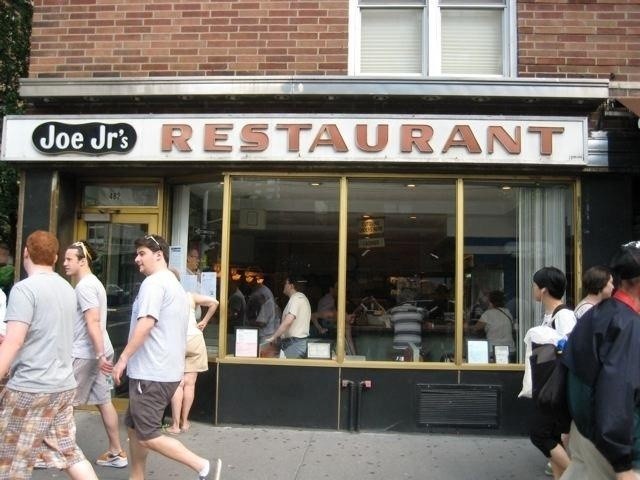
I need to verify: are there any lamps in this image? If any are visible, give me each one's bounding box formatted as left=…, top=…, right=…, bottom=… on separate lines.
left=213, top=266, right=263, bottom=283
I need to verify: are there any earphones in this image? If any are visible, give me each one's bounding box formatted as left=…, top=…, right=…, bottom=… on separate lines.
left=541, top=288, right=546, bottom=293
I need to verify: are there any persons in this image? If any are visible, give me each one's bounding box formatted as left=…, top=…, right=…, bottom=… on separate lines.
left=389, top=289, right=429, bottom=361
left=64, top=241, right=129, bottom=467
left=229, top=265, right=274, bottom=358
left=517, top=241, right=640, bottom=480
left=463, top=291, right=517, bottom=363
left=110, top=235, right=223, bottom=479
left=246, top=271, right=356, bottom=359
left=0, top=230, right=96, bottom=480
left=186, top=248, right=220, bottom=284
left=161, top=268, right=219, bottom=436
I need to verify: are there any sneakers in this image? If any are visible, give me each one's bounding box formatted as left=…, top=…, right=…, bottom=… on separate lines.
left=197, top=458, right=222, bottom=480
left=94, top=450, right=128, bottom=468
left=544, top=463, right=553, bottom=476
left=34, top=459, right=45, bottom=469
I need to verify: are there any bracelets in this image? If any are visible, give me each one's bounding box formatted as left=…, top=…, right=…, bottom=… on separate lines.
left=97, top=354, right=105, bottom=360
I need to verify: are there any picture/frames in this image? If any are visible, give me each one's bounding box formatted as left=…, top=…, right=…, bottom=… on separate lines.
left=493, top=345, right=510, bottom=364
left=234, top=326, right=259, bottom=358
left=306, top=341, right=332, bottom=360
left=466, top=339, right=490, bottom=362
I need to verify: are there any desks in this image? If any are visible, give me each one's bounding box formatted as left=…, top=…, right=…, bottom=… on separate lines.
left=350, top=324, right=484, bottom=362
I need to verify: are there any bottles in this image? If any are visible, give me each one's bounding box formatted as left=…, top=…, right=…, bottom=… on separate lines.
left=103, top=360, right=115, bottom=390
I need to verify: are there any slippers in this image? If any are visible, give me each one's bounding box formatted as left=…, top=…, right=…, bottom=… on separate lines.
left=162, top=422, right=191, bottom=435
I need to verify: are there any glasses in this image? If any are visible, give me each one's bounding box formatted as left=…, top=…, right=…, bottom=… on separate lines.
left=144, top=233, right=160, bottom=246
